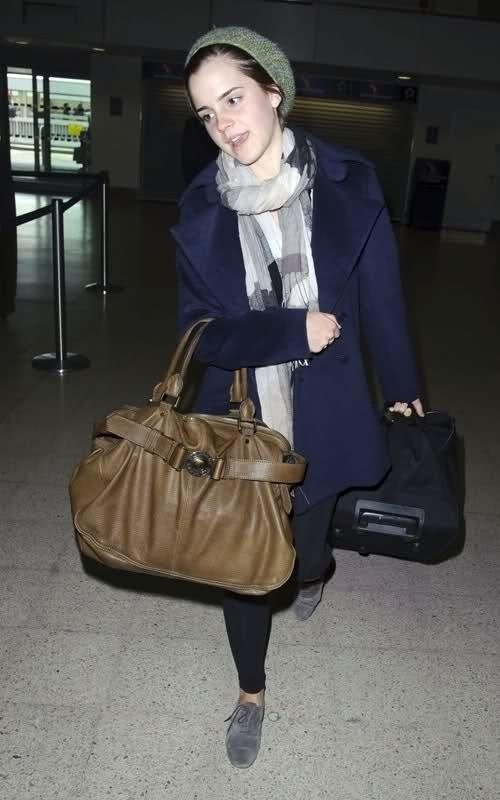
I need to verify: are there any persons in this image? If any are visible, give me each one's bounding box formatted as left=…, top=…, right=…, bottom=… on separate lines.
left=165, top=24, right=428, bottom=770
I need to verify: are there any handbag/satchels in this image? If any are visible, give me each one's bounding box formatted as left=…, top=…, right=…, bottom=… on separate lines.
left=67, top=403, right=307, bottom=598
left=329, top=409, right=465, bottom=565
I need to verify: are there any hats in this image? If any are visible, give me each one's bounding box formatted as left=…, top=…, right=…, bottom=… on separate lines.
left=185, top=27, right=297, bottom=122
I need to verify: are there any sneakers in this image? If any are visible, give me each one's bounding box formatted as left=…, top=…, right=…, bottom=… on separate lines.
left=293, top=580, right=327, bottom=620
left=225, top=684, right=268, bottom=768
